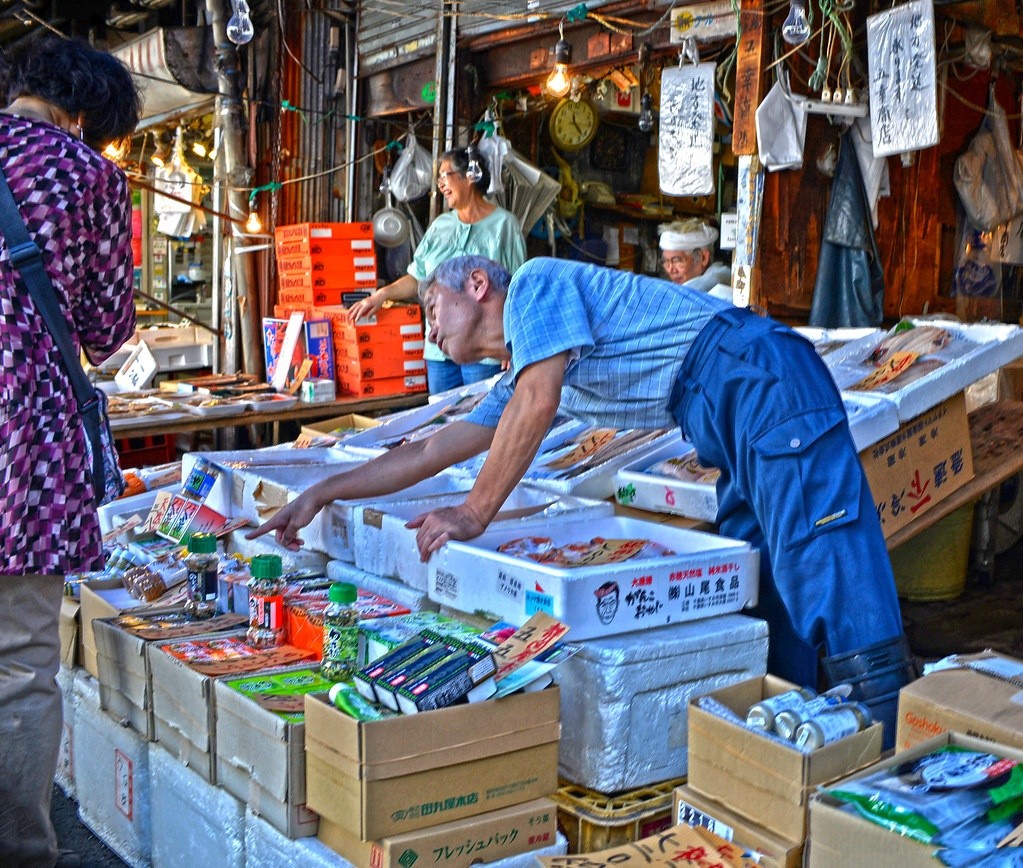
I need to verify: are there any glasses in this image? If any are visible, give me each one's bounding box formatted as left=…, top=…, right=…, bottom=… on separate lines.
left=436, top=170, right=460, bottom=184
left=659, top=249, right=699, bottom=268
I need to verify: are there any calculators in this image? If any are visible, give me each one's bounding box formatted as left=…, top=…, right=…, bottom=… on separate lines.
left=341, top=292, right=370, bottom=309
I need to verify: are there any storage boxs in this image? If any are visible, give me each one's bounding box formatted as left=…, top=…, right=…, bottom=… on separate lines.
left=618, top=222, right=634, bottom=272
left=53, top=318, right=1023, bottom=868
left=262, top=222, right=427, bottom=403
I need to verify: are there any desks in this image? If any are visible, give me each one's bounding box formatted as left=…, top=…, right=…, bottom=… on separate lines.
left=110, top=393, right=430, bottom=446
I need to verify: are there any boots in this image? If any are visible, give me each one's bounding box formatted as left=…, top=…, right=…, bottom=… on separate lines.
left=821, top=634, right=913, bottom=751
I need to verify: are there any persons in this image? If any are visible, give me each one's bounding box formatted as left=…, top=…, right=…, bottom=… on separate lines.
left=347, top=148, right=526, bottom=395
left=658, top=217, right=731, bottom=293
left=245, top=255, right=917, bottom=754
left=0, top=35, right=143, bottom=868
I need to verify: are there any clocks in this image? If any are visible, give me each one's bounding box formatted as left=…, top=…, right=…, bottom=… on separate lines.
left=549, top=96, right=599, bottom=151
left=591, top=123, right=632, bottom=171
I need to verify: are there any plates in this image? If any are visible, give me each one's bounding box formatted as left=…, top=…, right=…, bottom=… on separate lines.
left=246, top=392, right=298, bottom=412
left=180, top=400, right=247, bottom=416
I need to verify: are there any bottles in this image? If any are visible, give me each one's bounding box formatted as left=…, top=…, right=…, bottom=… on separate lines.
left=745, top=684, right=873, bottom=752
left=184, top=531, right=220, bottom=621
left=63, top=547, right=132, bottom=601
left=246, top=553, right=285, bottom=650
left=319, top=582, right=358, bottom=682
left=123, top=550, right=187, bottom=602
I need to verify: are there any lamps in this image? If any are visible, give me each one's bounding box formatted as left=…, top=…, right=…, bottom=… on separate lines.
left=151, top=127, right=174, bottom=166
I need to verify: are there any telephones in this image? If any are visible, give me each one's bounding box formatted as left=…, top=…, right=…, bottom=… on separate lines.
left=581, top=181, right=616, bottom=205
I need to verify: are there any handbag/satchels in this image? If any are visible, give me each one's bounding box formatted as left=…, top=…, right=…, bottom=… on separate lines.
left=77, top=389, right=126, bottom=507
left=389, top=112, right=432, bottom=202
